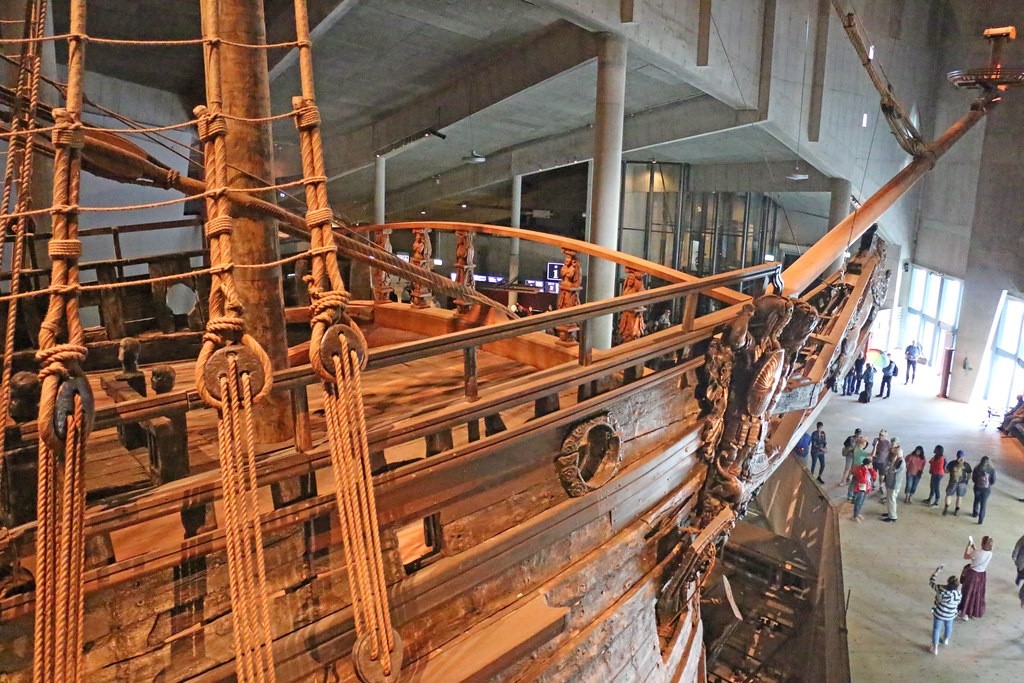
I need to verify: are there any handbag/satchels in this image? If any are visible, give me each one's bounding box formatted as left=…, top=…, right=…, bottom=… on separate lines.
left=960, top=564, right=971, bottom=584
left=842, top=447, right=849, bottom=456
left=858, top=484, right=866, bottom=492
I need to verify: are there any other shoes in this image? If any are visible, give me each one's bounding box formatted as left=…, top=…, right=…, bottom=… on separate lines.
left=940, top=634, right=948, bottom=645
left=904, top=496, right=913, bottom=503
left=921, top=499, right=939, bottom=509
left=930, top=647, right=937, bottom=655
left=943, top=508, right=949, bottom=515
left=883, top=517, right=897, bottom=522
left=839, top=477, right=888, bottom=504
left=997, top=427, right=1005, bottom=431
left=882, top=513, right=888, bottom=516
left=816, top=476, right=824, bottom=484
left=851, top=513, right=864, bottom=522
left=954, top=510, right=960, bottom=516
left=958, top=614, right=969, bottom=621
left=876, top=394, right=882, bottom=397
left=1002, top=429, right=1008, bottom=435
left=883, top=396, right=888, bottom=399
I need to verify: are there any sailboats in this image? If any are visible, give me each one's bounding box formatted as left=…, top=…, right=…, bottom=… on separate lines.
left=1, top=0, right=1024, bottom=683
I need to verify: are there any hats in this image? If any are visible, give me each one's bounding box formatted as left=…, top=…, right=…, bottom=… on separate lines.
left=957, top=450, right=964, bottom=457
left=855, top=428, right=861, bottom=434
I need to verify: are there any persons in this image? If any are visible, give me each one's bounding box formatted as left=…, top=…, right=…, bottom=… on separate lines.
left=928, top=567, right=963, bottom=655
left=643, top=309, right=671, bottom=336
left=453, top=236, right=475, bottom=287
left=958, top=535, right=993, bottom=621
left=412, top=233, right=426, bottom=259
left=1012, top=535, right=1024, bottom=608
left=511, top=302, right=556, bottom=337
left=795, top=422, right=828, bottom=484
left=839, top=350, right=896, bottom=403
left=839, top=428, right=997, bottom=524
left=557, top=254, right=582, bottom=309
left=719, top=294, right=794, bottom=478
left=618, top=273, right=645, bottom=341
left=904, top=340, right=920, bottom=384
left=997, top=395, right=1024, bottom=435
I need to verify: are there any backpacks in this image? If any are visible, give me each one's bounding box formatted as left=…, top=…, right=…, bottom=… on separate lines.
left=952, top=461, right=965, bottom=482
left=859, top=391, right=869, bottom=403
left=976, top=469, right=989, bottom=489
left=890, top=361, right=898, bottom=376
left=943, top=457, right=950, bottom=473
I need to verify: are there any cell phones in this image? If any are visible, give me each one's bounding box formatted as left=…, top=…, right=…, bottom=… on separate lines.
left=969, top=536, right=974, bottom=546
